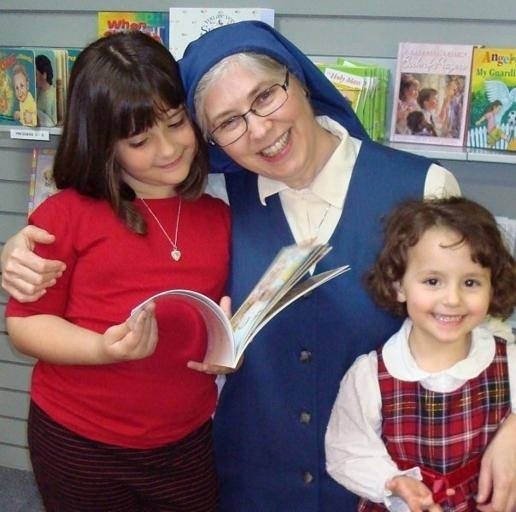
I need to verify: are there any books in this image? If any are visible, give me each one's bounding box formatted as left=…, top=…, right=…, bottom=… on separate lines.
left=313, top=42, right=516, bottom=152
left=0, top=8, right=275, bottom=127
left=130, top=236, right=352, bottom=369
left=26, top=147, right=62, bottom=219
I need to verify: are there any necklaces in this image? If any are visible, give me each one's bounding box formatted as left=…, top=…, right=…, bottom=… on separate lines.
left=132, top=186, right=182, bottom=261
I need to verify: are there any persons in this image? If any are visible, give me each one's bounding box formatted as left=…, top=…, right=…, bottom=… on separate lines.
left=0, top=20, right=516, bottom=512
left=3, top=29, right=234, bottom=512
left=324, top=196, right=516, bottom=512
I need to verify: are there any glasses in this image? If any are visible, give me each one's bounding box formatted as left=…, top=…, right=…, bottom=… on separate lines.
left=208, top=70, right=290, bottom=149
